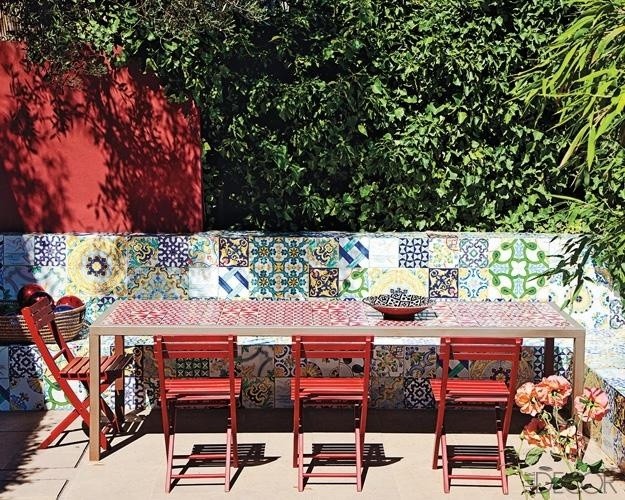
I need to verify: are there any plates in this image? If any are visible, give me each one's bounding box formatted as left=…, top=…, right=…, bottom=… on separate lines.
left=362, top=295, right=436, bottom=314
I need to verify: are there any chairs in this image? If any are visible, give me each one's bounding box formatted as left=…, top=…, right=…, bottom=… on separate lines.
left=429, top=332, right=524, bottom=494
left=289, top=334, right=374, bottom=490
left=152, top=331, right=241, bottom=491
left=22, top=297, right=131, bottom=452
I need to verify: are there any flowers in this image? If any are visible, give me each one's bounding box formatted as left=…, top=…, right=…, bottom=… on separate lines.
left=504, top=373, right=610, bottom=500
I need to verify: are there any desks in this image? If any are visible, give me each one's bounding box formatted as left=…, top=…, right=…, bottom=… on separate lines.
left=89, top=297, right=585, bottom=460
